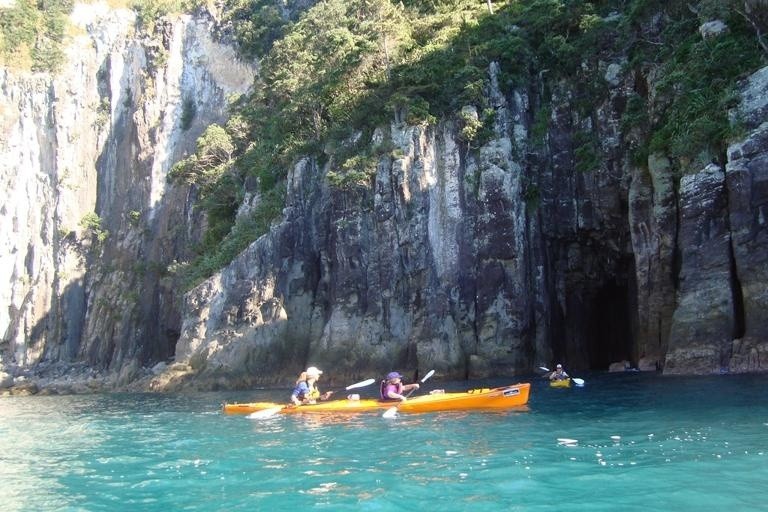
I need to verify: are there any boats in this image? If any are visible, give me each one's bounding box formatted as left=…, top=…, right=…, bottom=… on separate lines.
left=550, top=378, right=571, bottom=388
left=222, top=383, right=532, bottom=415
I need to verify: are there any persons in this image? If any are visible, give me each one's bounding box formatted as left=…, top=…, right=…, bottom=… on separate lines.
left=380, top=372, right=420, bottom=401
left=549, top=364, right=569, bottom=381
left=291, top=367, right=333, bottom=406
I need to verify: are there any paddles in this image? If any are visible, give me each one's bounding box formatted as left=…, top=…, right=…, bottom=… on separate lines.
left=245, top=378, right=375, bottom=418
left=539, top=367, right=585, bottom=385
left=383, top=370, right=435, bottom=418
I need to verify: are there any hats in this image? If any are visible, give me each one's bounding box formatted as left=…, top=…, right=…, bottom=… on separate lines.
left=307, top=367, right=323, bottom=376
left=386, top=371, right=403, bottom=380
